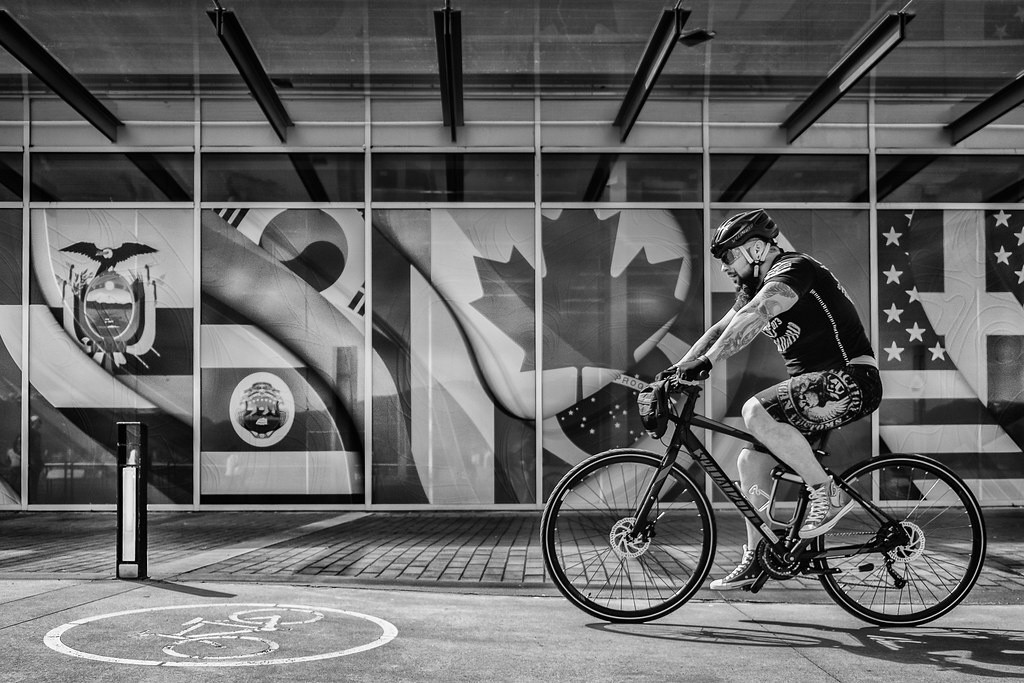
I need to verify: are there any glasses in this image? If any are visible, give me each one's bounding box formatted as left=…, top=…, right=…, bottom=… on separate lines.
left=721, top=246, right=751, bottom=265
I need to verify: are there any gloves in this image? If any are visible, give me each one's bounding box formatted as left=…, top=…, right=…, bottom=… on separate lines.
left=676, top=355, right=713, bottom=382
left=654, top=370, right=669, bottom=381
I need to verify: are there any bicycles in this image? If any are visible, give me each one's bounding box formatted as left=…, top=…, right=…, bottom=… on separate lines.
left=540, top=370, right=987, bottom=627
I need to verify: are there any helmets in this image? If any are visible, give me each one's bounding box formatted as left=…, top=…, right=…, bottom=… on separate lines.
left=709, top=209, right=781, bottom=255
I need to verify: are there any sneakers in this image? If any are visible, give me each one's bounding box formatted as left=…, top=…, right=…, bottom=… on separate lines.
left=711, top=544, right=763, bottom=590
left=798, top=475, right=857, bottom=539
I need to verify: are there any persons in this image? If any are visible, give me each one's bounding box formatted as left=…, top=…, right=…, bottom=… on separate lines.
left=655, top=209, right=883, bottom=590
